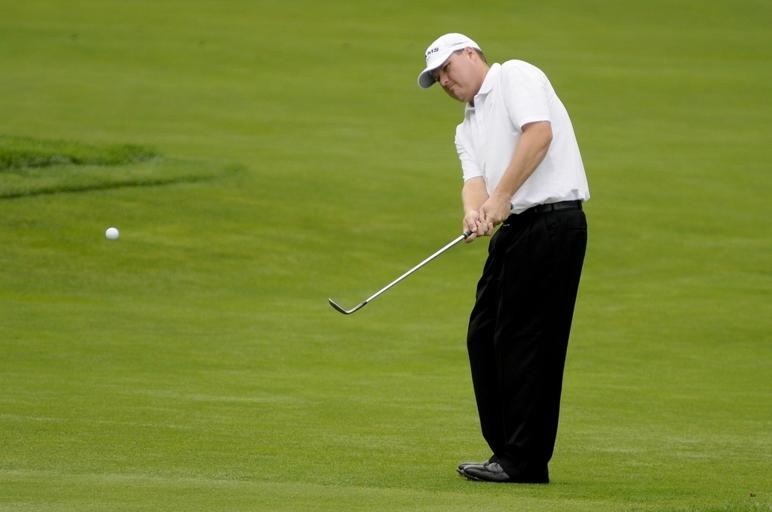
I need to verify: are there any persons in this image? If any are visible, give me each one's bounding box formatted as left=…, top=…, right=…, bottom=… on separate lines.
left=418, top=32, right=591, bottom=483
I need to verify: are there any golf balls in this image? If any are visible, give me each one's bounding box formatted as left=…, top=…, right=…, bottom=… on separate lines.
left=105, top=227, right=119, bottom=241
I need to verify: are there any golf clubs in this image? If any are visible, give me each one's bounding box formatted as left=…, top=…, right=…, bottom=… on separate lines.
left=328, top=231, right=472, bottom=314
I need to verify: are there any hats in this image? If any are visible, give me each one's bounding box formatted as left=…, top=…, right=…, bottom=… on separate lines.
left=418, top=32, right=481, bottom=90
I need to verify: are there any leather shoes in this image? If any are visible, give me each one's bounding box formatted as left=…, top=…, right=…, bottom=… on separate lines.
left=456, top=459, right=549, bottom=482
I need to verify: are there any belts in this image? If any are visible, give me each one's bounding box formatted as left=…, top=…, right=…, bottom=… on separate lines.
left=502, top=201, right=581, bottom=228
